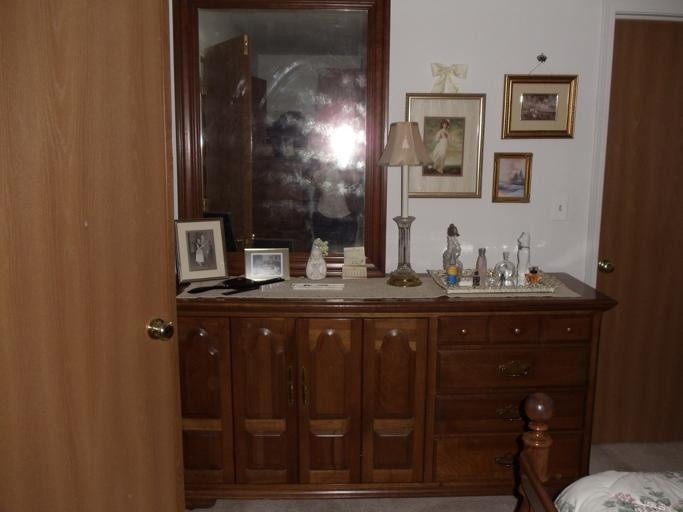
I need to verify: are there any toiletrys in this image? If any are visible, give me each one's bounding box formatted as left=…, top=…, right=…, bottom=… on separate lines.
left=446, top=244, right=544, bottom=289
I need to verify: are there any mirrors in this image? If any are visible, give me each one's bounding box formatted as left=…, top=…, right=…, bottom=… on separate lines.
left=172, top=1, right=393, bottom=277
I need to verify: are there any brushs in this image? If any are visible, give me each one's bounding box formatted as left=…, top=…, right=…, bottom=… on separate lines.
left=187, top=278, right=255, bottom=294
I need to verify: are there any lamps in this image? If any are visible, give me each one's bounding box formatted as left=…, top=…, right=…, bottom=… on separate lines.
left=378, top=121, right=434, bottom=286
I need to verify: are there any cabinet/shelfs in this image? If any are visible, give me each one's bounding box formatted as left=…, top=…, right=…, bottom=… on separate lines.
left=304, top=273, right=435, bottom=496
left=178, top=272, right=302, bottom=497
left=427, top=273, right=618, bottom=501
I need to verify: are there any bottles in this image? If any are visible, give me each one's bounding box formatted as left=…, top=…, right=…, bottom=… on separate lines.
left=446, top=232, right=530, bottom=287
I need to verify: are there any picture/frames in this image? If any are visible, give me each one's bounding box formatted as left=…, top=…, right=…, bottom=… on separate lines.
left=243, top=246, right=291, bottom=282
left=171, top=216, right=228, bottom=284
left=404, top=72, right=578, bottom=205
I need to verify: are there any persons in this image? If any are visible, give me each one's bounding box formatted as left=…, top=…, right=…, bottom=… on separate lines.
left=199, top=234, right=210, bottom=267
left=195, top=238, right=204, bottom=267
left=432, top=118, right=450, bottom=173
left=310, top=136, right=361, bottom=251
